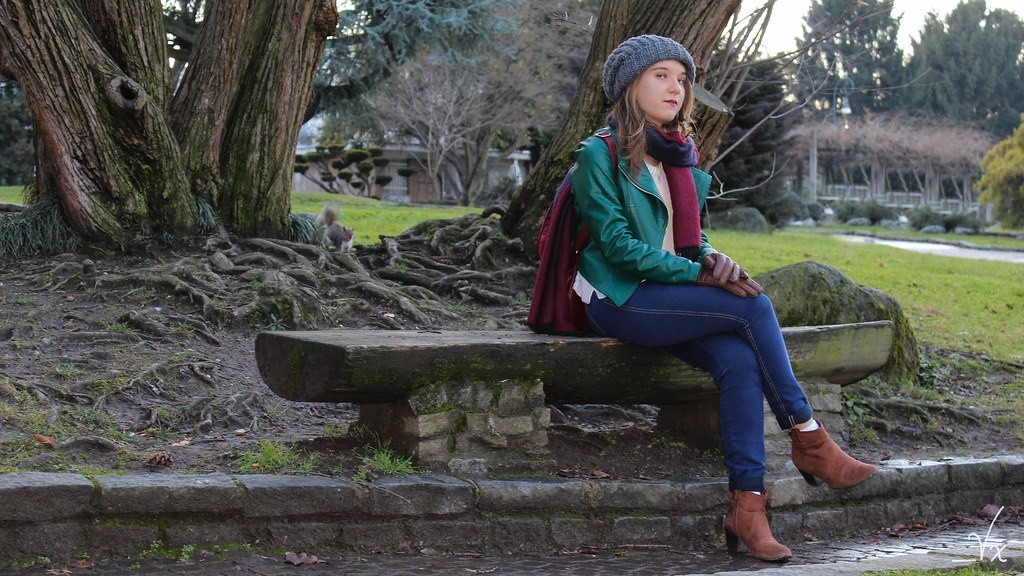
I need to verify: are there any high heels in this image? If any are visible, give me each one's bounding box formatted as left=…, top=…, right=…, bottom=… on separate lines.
left=789, top=423, right=880, bottom=491
left=723, top=488, right=795, bottom=563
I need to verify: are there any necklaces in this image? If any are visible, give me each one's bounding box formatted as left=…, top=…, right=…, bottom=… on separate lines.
left=656, top=165, right=663, bottom=184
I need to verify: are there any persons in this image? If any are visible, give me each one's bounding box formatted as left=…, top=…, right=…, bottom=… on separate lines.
left=525, top=32, right=879, bottom=562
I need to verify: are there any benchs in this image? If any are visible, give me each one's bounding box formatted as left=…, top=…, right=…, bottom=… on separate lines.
left=254, top=320, right=897, bottom=465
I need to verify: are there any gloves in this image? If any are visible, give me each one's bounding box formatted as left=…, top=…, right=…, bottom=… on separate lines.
left=704, top=251, right=751, bottom=286
left=699, top=257, right=764, bottom=300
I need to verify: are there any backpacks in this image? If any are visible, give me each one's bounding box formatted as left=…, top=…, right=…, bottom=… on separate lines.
left=529, top=123, right=619, bottom=335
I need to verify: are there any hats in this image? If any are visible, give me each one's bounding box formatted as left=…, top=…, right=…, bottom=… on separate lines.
left=601, top=33, right=696, bottom=102
